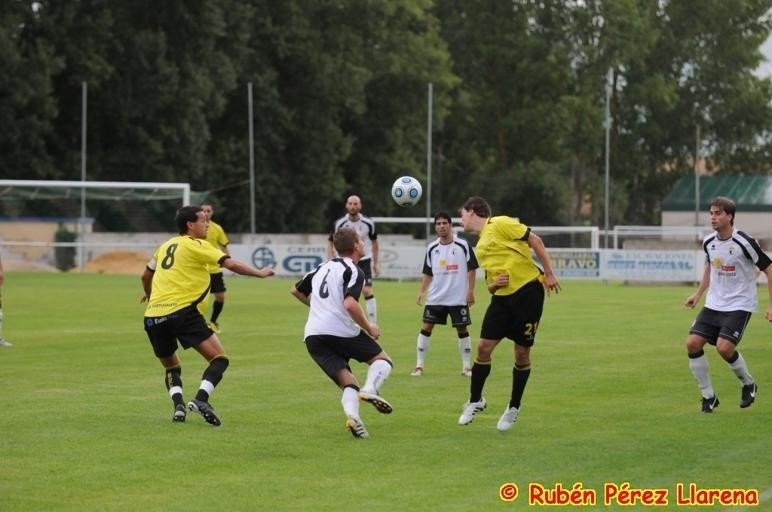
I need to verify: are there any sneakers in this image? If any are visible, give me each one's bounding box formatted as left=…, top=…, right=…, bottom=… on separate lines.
left=358, top=390, right=393, bottom=416
left=739, top=382, right=758, bottom=408
left=462, top=367, right=473, bottom=376
left=188, top=399, right=222, bottom=427
left=496, top=404, right=522, bottom=431
left=458, top=396, right=488, bottom=426
left=702, top=394, right=720, bottom=413
left=209, top=320, right=223, bottom=335
left=345, top=418, right=370, bottom=439
left=172, top=403, right=188, bottom=422
left=411, top=366, right=424, bottom=376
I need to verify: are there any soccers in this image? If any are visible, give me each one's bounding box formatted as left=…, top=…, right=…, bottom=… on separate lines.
left=391, top=176, right=422, bottom=207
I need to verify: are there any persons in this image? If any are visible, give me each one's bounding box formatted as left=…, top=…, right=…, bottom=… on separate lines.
left=289, top=227, right=393, bottom=442
left=198, top=202, right=231, bottom=330
left=455, top=195, right=563, bottom=434
left=329, top=194, right=383, bottom=329
left=142, top=205, right=275, bottom=423
left=411, top=212, right=474, bottom=376
left=684, top=196, right=770, bottom=412
